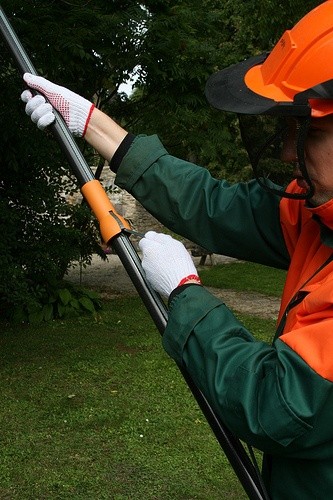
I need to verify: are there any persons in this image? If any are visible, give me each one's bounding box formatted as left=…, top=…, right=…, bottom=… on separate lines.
left=21, top=0, right=333, bottom=500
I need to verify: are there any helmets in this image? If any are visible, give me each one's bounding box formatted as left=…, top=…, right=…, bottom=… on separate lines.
left=205, top=0, right=333, bottom=119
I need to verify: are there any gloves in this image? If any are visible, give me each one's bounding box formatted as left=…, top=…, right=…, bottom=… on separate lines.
left=20, top=73, right=95, bottom=139
left=139, top=232, right=202, bottom=301
